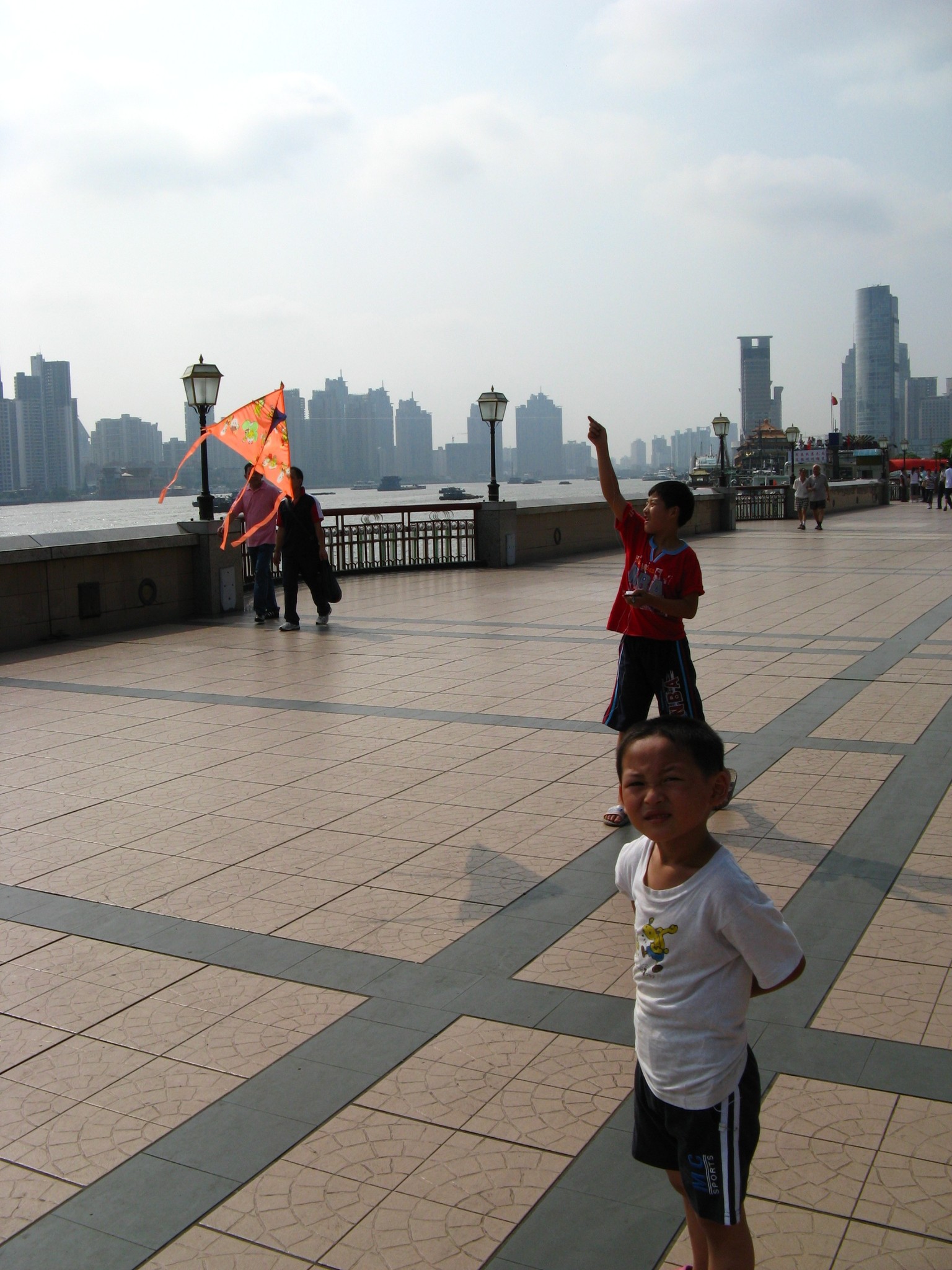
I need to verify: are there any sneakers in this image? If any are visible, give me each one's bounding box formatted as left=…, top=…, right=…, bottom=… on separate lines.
left=315, top=606, right=332, bottom=625
left=279, top=621, right=301, bottom=631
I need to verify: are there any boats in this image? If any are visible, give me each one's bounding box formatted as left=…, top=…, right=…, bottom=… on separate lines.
left=642, top=461, right=678, bottom=480
left=439, top=486, right=484, bottom=501
left=507, top=477, right=543, bottom=484
left=584, top=475, right=630, bottom=481
left=684, top=444, right=730, bottom=490
left=350, top=475, right=426, bottom=491
left=558, top=481, right=572, bottom=484
left=0, top=490, right=29, bottom=506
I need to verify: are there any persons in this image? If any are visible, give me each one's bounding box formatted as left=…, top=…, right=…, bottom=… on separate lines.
left=272, top=466, right=333, bottom=631
left=586, top=414, right=739, bottom=826
left=899, top=462, right=952, bottom=511
left=217, top=462, right=281, bottom=623
left=792, top=464, right=830, bottom=530
left=615, top=716, right=807, bottom=1270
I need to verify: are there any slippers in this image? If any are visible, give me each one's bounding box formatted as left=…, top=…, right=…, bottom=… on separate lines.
left=710, top=768, right=737, bottom=811
left=602, top=805, right=630, bottom=827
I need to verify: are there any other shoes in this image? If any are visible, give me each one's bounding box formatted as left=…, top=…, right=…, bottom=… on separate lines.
left=818, top=526, right=822, bottom=530
left=798, top=524, right=802, bottom=529
left=943, top=506, right=947, bottom=511
left=254, top=613, right=268, bottom=624
left=264, top=610, right=280, bottom=619
left=936, top=507, right=942, bottom=509
left=814, top=525, right=819, bottom=529
left=927, top=506, right=932, bottom=509
left=801, top=525, right=805, bottom=529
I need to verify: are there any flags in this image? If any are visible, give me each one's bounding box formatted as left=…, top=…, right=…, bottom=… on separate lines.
left=831, top=396, right=838, bottom=405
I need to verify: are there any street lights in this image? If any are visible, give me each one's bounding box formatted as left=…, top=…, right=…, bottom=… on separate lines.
left=785, top=423, right=800, bottom=485
left=475, top=385, right=510, bottom=501
left=900, top=438, right=909, bottom=471
left=878, top=435, right=888, bottom=479
left=711, top=412, right=730, bottom=487
left=178, top=353, right=224, bottom=520
left=933, top=443, right=944, bottom=469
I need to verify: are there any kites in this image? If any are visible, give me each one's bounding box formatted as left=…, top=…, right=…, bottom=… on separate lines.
left=157, top=381, right=294, bottom=550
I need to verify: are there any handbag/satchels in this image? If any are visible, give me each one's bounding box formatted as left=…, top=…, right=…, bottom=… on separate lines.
left=321, top=559, right=342, bottom=603
